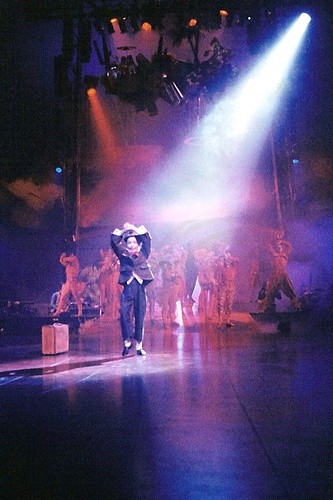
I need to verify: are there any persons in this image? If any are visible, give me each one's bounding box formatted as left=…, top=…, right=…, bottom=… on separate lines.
left=112, top=222, right=153, bottom=357
left=52, top=244, right=85, bottom=323
left=82, top=227, right=308, bottom=330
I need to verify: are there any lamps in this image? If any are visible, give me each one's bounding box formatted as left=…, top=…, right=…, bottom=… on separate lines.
left=85, top=1, right=258, bottom=105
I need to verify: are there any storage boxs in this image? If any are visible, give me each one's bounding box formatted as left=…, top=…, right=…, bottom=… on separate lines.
left=42, top=323, right=70, bottom=356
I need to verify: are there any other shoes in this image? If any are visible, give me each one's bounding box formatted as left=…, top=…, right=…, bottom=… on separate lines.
left=122, top=342, right=134, bottom=357
left=137, top=349, right=147, bottom=357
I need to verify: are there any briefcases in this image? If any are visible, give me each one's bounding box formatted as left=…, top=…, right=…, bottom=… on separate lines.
left=41, top=322, right=70, bottom=356
